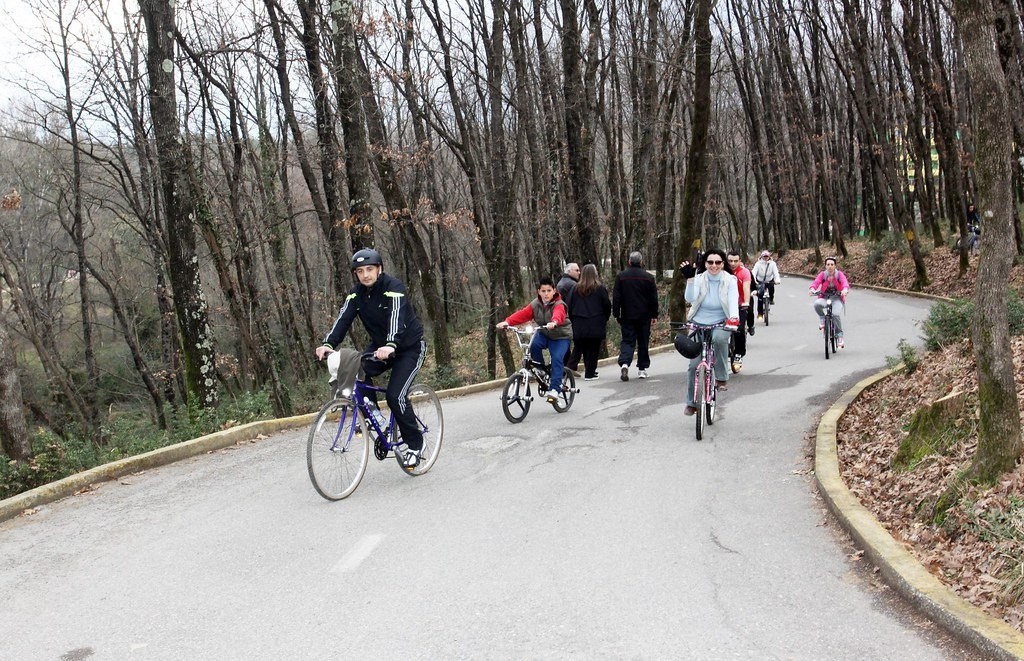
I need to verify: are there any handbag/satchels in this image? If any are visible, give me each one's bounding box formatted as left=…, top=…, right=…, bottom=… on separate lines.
left=756, top=284, right=765, bottom=293
left=581, top=326, right=609, bottom=363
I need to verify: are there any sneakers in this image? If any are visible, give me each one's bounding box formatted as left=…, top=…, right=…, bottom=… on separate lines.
left=620, top=364, right=629, bottom=382
left=530, top=367, right=545, bottom=376
left=734, top=354, right=743, bottom=363
left=638, top=369, right=650, bottom=379
left=585, top=372, right=599, bottom=380
left=355, top=418, right=376, bottom=430
left=837, top=337, right=844, bottom=346
left=820, top=323, right=825, bottom=328
left=403, top=439, right=426, bottom=467
left=547, top=389, right=559, bottom=402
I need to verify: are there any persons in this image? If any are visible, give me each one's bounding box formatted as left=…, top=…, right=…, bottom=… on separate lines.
left=678, top=248, right=740, bottom=417
left=612, top=251, right=658, bottom=381
left=808, top=258, right=849, bottom=347
left=556, top=263, right=612, bottom=380
left=315, top=248, right=428, bottom=468
left=751, top=250, right=781, bottom=321
left=727, top=251, right=758, bottom=360
left=495, top=276, right=573, bottom=402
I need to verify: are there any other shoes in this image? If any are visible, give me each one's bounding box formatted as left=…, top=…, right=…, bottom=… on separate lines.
left=717, top=381, right=726, bottom=391
left=685, top=406, right=695, bottom=415
left=770, top=301, right=774, bottom=305
left=572, top=370, right=581, bottom=377
left=749, top=327, right=754, bottom=335
left=758, top=314, right=763, bottom=320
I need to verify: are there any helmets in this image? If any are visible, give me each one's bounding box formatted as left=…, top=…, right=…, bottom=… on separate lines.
left=674, top=333, right=703, bottom=359
left=350, top=247, right=384, bottom=270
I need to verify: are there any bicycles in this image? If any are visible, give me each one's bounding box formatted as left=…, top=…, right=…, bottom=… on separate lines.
left=501, top=325, right=580, bottom=424
left=684, top=322, right=726, bottom=439
left=728, top=306, right=744, bottom=373
left=756, top=281, right=775, bottom=326
left=813, top=291, right=842, bottom=360
left=306, top=353, right=444, bottom=502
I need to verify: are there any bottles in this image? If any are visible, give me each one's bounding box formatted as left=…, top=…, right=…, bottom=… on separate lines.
left=364, top=396, right=386, bottom=427
left=538, top=370, right=549, bottom=380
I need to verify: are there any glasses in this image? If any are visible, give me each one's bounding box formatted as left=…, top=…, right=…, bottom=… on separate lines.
left=706, top=260, right=724, bottom=265
left=764, top=256, right=768, bottom=257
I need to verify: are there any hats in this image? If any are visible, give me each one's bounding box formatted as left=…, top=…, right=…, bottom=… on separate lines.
left=762, top=250, right=770, bottom=257
